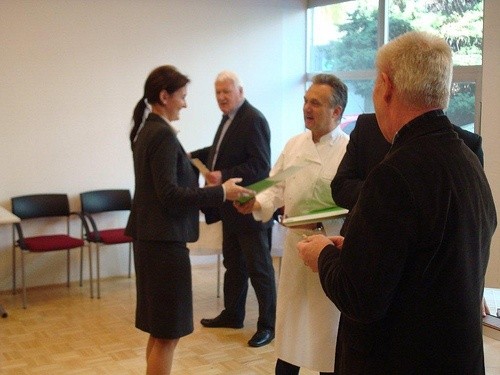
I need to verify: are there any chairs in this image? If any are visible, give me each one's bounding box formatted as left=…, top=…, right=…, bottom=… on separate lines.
left=78, top=190, right=135, bottom=299
left=14, top=192, right=93, bottom=309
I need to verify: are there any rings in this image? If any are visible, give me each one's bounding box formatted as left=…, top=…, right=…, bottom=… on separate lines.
left=304, top=262, right=307, bottom=266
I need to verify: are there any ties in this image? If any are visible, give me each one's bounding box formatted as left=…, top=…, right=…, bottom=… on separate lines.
left=204, top=114, right=230, bottom=187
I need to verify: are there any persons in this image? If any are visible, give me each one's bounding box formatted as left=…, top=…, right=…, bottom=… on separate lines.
left=297, top=31, right=497, bottom=375
left=233, top=75, right=351, bottom=375
left=186, top=70, right=277, bottom=348
left=125, top=65, right=256, bottom=375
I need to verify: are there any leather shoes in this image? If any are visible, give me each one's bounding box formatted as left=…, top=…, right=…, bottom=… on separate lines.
left=247, top=327, right=275, bottom=347
left=200, top=314, right=244, bottom=329
